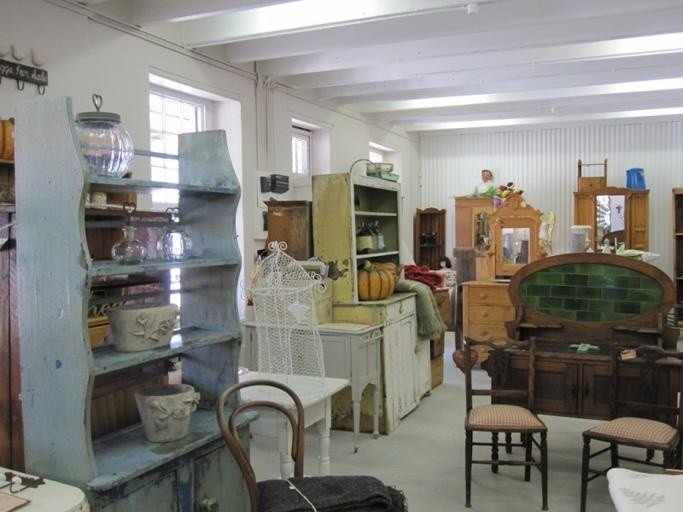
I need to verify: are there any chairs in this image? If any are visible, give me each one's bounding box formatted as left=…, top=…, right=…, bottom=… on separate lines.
left=579, top=339, right=682, bottom=512
left=217, top=380, right=408, bottom=512
left=463, top=337, right=549, bottom=510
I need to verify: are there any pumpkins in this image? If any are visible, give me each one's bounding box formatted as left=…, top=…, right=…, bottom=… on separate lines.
left=359, top=260, right=395, bottom=300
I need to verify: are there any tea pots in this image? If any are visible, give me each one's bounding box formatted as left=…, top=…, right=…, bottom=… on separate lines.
left=626, top=168, right=646, bottom=189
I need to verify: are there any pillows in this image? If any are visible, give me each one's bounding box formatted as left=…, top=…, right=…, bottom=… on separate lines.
left=605, top=468, right=682, bottom=512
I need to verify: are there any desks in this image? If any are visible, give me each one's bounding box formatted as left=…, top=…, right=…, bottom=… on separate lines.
left=242, top=321, right=383, bottom=455
left=0, top=467, right=90, bottom=511
left=239, top=372, right=350, bottom=481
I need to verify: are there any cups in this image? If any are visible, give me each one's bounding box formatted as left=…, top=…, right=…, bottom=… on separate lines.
left=85, top=193, right=89, bottom=204
left=91, top=191, right=106, bottom=210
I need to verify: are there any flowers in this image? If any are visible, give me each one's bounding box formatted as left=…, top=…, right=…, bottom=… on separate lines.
left=482, top=181, right=524, bottom=210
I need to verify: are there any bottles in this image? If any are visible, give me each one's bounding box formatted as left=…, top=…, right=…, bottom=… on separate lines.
left=154, top=219, right=193, bottom=261
left=356, top=220, right=385, bottom=254
left=110, top=225, right=147, bottom=266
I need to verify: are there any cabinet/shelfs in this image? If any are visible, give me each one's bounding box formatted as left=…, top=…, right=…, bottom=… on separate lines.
left=573, top=189, right=651, bottom=259
left=266, top=197, right=311, bottom=262
left=454, top=197, right=494, bottom=249
left=491, top=350, right=683, bottom=425
left=429, top=288, right=450, bottom=392
left=12, top=98, right=260, bottom=510
left=461, top=282, right=513, bottom=365
left=414, top=207, right=447, bottom=270
left=671, top=188, right=683, bottom=316
left=311, top=173, right=431, bottom=418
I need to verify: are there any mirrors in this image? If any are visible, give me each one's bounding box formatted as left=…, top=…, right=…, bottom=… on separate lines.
left=594, top=191, right=628, bottom=251
left=490, top=205, right=543, bottom=279
